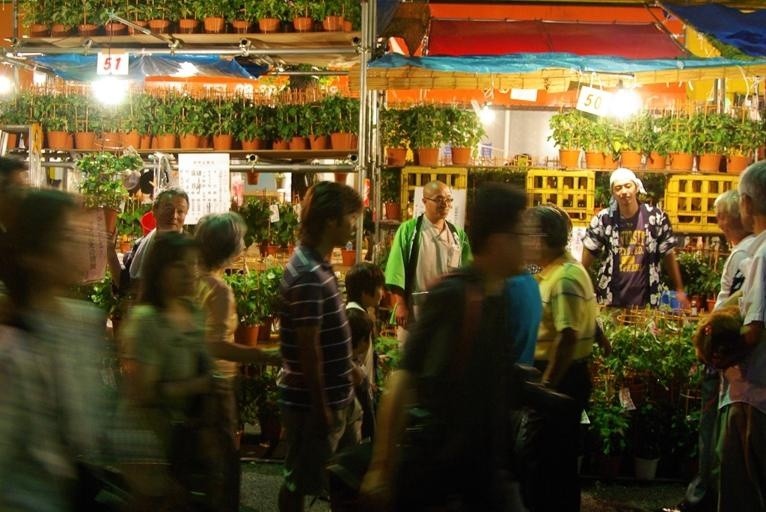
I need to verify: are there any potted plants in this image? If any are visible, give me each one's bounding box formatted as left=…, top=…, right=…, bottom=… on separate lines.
left=1, top=94, right=359, bottom=152
left=402, top=103, right=449, bottom=166
left=118, top=200, right=155, bottom=254
left=341, top=207, right=373, bottom=266
left=367, top=166, right=402, bottom=221
left=223, top=264, right=287, bottom=347
left=677, top=250, right=723, bottom=312
left=545, top=111, right=766, bottom=174
left=20, top=1, right=360, bottom=39
left=376, top=105, right=409, bottom=167
left=231, top=194, right=301, bottom=257
left=76, top=152, right=145, bottom=237
left=592, top=303, right=720, bottom=456
left=447, top=107, right=486, bottom=166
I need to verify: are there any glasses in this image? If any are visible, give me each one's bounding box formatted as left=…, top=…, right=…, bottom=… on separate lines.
left=424, top=197, right=454, bottom=203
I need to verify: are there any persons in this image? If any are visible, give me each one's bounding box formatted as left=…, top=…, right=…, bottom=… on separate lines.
left=342, top=260, right=385, bottom=424
left=342, top=307, right=374, bottom=366
left=382, top=179, right=474, bottom=354
left=660, top=188, right=754, bottom=512
left=0, top=150, right=34, bottom=204
left=0, top=187, right=118, bottom=512
left=578, top=166, right=691, bottom=311
left=270, top=178, right=365, bottom=512
left=117, top=227, right=241, bottom=510
left=101, top=183, right=191, bottom=335
left=707, top=159, right=765, bottom=511
left=526, top=202, right=612, bottom=512
left=192, top=209, right=282, bottom=511
left=352, top=174, right=541, bottom=512
